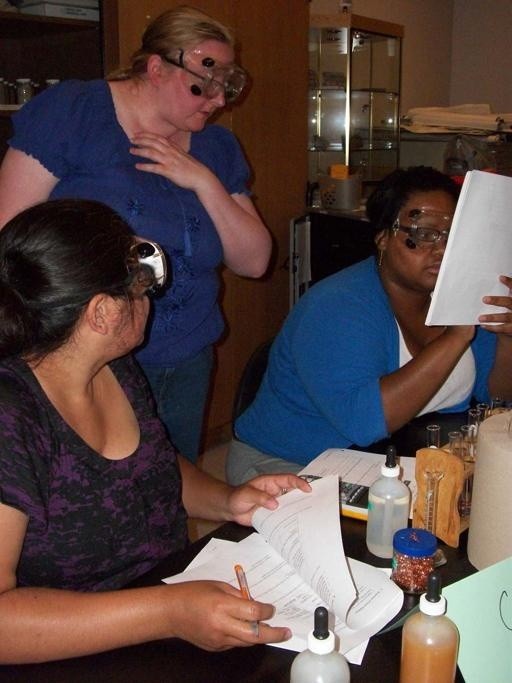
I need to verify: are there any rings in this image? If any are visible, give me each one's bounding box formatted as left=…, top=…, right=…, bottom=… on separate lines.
left=282, top=487, right=287, bottom=495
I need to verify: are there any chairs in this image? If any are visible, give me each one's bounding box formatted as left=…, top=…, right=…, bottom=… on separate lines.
left=233, top=338, right=272, bottom=436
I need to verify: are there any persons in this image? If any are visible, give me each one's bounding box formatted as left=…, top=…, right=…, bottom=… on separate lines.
left=225, top=159, right=511, bottom=514
left=0, top=195, right=313, bottom=664
left=1, top=6, right=275, bottom=463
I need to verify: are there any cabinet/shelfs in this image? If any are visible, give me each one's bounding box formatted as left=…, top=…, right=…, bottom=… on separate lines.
left=307, top=15, right=406, bottom=213
left=0, top=0, right=104, bottom=114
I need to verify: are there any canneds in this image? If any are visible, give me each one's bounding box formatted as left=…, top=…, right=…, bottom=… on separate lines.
left=0, top=78, right=61, bottom=104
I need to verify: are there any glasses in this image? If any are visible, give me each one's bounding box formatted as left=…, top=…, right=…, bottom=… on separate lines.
left=393, top=224, right=448, bottom=243
left=165, top=48, right=247, bottom=104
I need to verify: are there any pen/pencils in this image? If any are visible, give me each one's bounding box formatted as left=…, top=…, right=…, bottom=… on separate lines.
left=234, top=566, right=259, bottom=637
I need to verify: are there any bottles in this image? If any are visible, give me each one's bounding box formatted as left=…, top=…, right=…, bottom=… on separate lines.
left=288, top=605, right=352, bottom=683
left=364, top=446, right=410, bottom=561
left=397, top=572, right=460, bottom=681
left=427, top=395, right=506, bottom=513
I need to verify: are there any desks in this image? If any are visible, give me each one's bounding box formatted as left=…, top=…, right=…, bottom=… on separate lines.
left=0, top=398, right=512, bottom=681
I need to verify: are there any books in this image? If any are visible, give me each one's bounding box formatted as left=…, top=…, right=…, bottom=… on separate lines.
left=160, top=473, right=407, bottom=666
left=295, top=446, right=418, bottom=528
left=424, top=168, right=512, bottom=328
